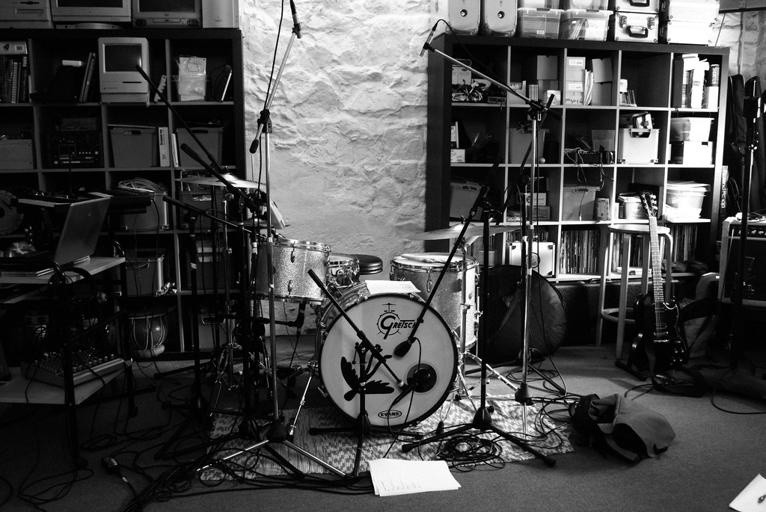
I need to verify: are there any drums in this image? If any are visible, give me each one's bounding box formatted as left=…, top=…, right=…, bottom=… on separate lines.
left=390, top=252, right=477, bottom=348
left=327, top=255, right=358, bottom=288
left=251, top=235, right=329, bottom=305
left=316, top=280, right=462, bottom=430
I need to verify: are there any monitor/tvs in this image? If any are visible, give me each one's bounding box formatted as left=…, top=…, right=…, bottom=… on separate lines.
left=132, top=0, right=201, bottom=28
left=98, top=37, right=150, bottom=94
left=50, top=0, right=131, bottom=29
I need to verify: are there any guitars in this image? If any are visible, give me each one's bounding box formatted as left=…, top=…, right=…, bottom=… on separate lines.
left=630, top=192, right=689, bottom=377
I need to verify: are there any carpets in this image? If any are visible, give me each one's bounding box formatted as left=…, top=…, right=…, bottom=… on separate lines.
left=200, top=393, right=574, bottom=481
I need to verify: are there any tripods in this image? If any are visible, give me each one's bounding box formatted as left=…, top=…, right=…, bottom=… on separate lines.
left=308, top=269, right=423, bottom=478
left=159, top=25, right=354, bottom=488
left=689, top=80, right=766, bottom=377
left=402, top=155, right=557, bottom=468
left=423, top=43, right=578, bottom=441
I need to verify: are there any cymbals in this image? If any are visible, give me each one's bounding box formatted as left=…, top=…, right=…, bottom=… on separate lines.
left=174, top=173, right=266, bottom=190
left=404, top=223, right=523, bottom=241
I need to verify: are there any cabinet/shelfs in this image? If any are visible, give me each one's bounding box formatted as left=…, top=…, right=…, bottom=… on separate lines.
left=424, top=29, right=766, bottom=350
left=0, top=28, right=251, bottom=361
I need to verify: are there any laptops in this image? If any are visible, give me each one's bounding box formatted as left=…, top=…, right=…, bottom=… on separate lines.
left=0, top=197, right=111, bottom=270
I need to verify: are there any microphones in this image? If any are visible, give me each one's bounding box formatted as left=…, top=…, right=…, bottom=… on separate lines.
left=290, top=0, right=302, bottom=38
left=419, top=23, right=437, bottom=57
left=394, top=337, right=416, bottom=358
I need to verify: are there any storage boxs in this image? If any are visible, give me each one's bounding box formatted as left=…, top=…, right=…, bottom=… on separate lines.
left=517, top=0, right=722, bottom=44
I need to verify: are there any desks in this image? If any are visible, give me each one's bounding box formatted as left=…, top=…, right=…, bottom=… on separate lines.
left=1, top=256, right=139, bottom=466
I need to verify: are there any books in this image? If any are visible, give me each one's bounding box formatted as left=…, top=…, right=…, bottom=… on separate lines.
left=559, top=229, right=599, bottom=275
left=665, top=224, right=705, bottom=263
